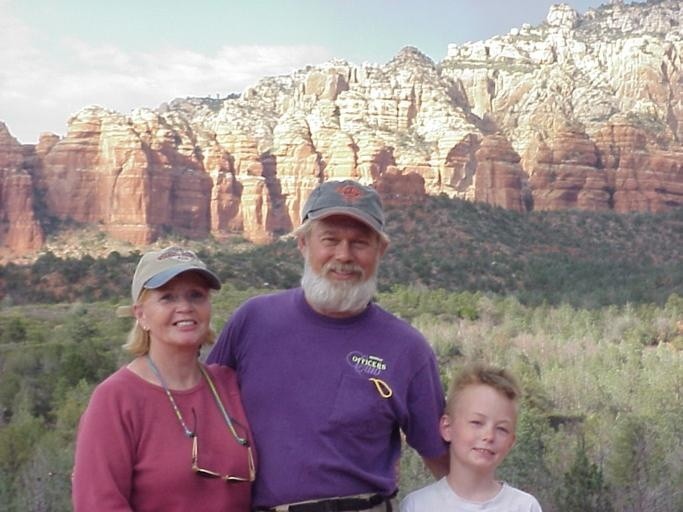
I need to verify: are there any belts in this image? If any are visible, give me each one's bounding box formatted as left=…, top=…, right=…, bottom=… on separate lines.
left=253, top=487, right=399, bottom=512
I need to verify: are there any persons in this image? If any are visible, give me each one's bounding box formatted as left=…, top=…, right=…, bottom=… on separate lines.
left=387, top=360, right=544, bottom=511
left=66, top=243, right=261, bottom=512
left=196, top=176, right=451, bottom=511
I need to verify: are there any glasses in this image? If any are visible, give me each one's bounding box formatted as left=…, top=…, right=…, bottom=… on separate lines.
left=192, top=434, right=257, bottom=483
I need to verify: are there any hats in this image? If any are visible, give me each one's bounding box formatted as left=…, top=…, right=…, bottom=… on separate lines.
left=131, top=246, right=222, bottom=303
left=302, top=179, right=385, bottom=235
